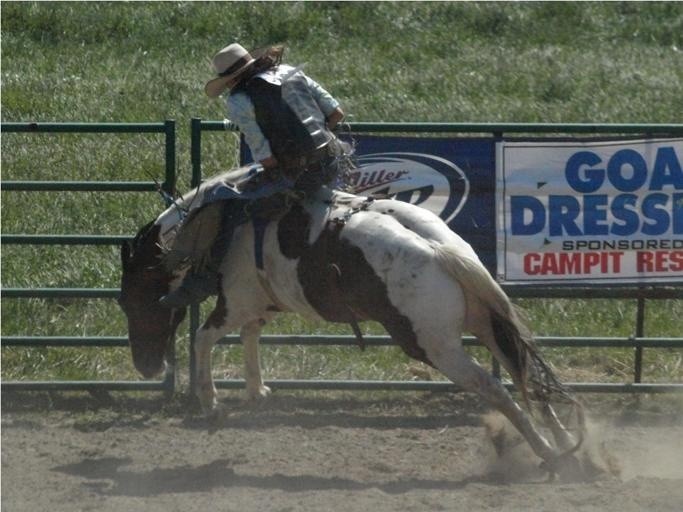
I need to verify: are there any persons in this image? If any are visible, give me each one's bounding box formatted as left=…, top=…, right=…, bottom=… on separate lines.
left=157, top=42, right=344, bottom=309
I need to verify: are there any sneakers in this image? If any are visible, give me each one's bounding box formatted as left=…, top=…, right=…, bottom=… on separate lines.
left=159, top=273, right=215, bottom=305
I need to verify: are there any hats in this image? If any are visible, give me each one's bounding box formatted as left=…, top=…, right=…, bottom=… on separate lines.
left=205, top=42, right=267, bottom=97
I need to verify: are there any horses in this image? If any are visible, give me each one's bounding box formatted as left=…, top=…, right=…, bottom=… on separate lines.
left=115, top=162, right=612, bottom=487
